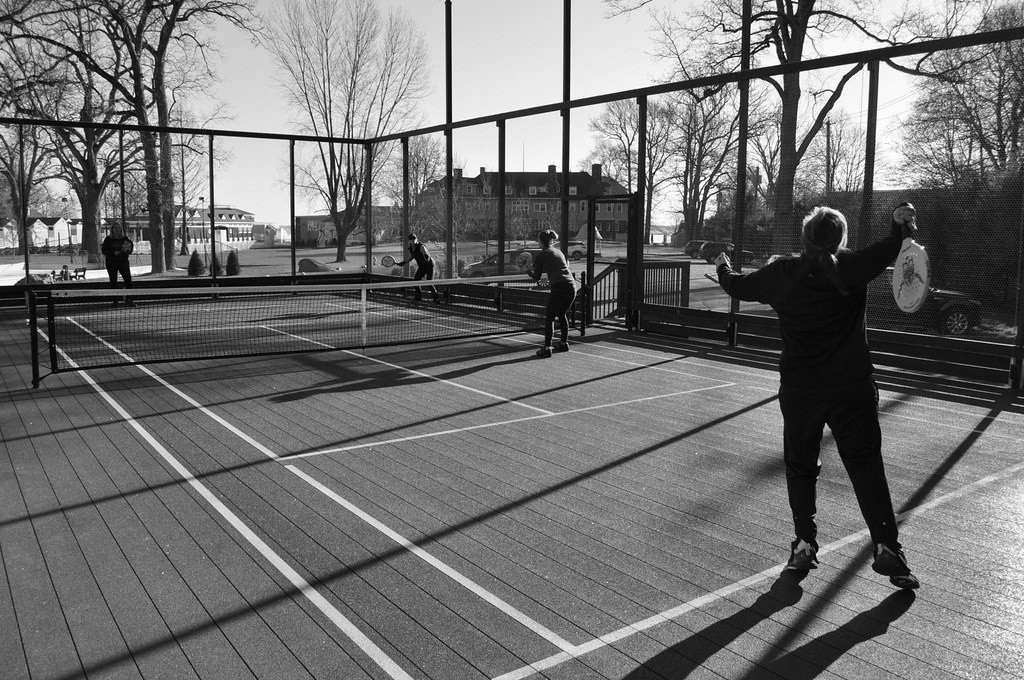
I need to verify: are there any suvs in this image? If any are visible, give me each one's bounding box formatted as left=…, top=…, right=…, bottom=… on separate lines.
left=699, top=241, right=753, bottom=265
left=684, top=240, right=708, bottom=259
left=549, top=240, right=588, bottom=260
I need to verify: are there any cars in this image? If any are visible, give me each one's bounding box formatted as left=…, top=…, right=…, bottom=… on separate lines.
left=866, top=265, right=985, bottom=338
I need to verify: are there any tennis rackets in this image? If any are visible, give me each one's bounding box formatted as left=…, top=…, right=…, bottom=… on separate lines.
left=119, top=239, right=134, bottom=255
left=892, top=220, right=932, bottom=313
left=514, top=251, right=538, bottom=280
left=381, top=255, right=400, bottom=268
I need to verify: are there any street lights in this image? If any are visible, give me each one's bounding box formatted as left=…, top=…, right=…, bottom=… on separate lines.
left=61, top=197, right=74, bottom=263
left=199, top=196, right=209, bottom=268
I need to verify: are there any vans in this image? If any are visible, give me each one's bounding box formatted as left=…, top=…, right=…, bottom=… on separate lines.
left=459, top=248, right=571, bottom=289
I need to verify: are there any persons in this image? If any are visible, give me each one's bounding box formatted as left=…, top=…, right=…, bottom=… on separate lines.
left=523, top=230, right=576, bottom=358
left=715, top=202, right=920, bottom=588
left=399, top=234, right=441, bottom=306
left=101, top=223, right=137, bottom=307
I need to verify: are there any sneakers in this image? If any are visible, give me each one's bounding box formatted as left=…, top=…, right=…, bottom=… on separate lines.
left=788, top=538, right=820, bottom=571
left=872, top=540, right=920, bottom=589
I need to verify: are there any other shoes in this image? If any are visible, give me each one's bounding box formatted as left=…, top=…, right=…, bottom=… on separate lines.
left=433, top=297, right=440, bottom=301
left=414, top=296, right=423, bottom=300
left=536, top=346, right=551, bottom=358
left=554, top=342, right=568, bottom=352
left=125, top=302, right=137, bottom=307
left=114, top=304, right=121, bottom=308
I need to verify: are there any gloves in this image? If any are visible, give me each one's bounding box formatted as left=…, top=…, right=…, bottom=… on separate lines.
left=397, top=261, right=406, bottom=266
left=424, top=253, right=428, bottom=259
